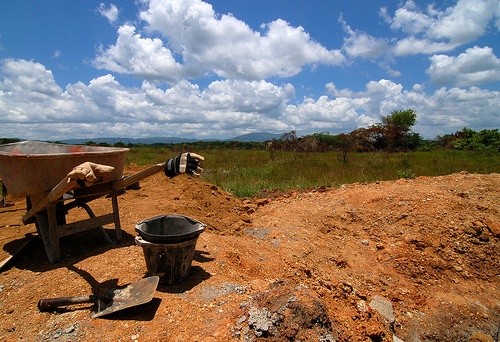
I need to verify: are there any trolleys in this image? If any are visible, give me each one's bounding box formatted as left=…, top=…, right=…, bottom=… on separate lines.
left=0, top=139, right=204, bottom=262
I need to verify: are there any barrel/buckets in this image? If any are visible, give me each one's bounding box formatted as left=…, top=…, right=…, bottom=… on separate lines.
left=134, top=213, right=207, bottom=286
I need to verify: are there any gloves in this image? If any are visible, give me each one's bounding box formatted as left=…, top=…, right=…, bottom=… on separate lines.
left=68, top=162, right=115, bottom=189
left=164, top=152, right=205, bottom=178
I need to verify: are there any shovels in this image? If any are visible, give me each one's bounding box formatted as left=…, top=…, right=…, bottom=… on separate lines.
left=38, top=275, right=159, bottom=319
